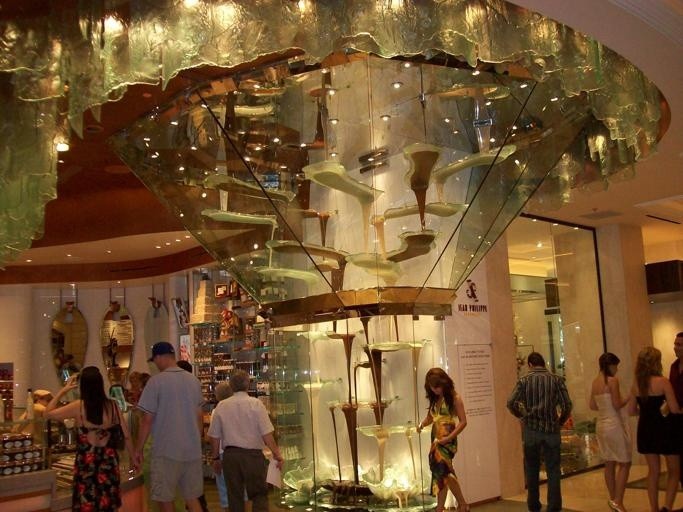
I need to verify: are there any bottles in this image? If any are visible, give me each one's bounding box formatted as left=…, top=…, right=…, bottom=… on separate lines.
left=25, top=388, right=35, bottom=420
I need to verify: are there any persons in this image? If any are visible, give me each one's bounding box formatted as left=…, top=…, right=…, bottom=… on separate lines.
left=175, top=360, right=210, bottom=512
left=667, top=331, right=682, bottom=511
left=42, top=366, right=143, bottom=511
left=207, top=382, right=249, bottom=511
left=628, top=346, right=682, bottom=512
left=588, top=351, right=632, bottom=512
left=10, top=389, right=58, bottom=449
left=414, top=367, right=471, bottom=512
left=108, top=370, right=151, bottom=406
left=505, top=351, right=573, bottom=512
left=131, top=341, right=206, bottom=511
left=207, top=367, right=284, bottom=511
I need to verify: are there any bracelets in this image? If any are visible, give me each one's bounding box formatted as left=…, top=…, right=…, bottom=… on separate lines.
left=211, top=455, right=219, bottom=461
left=418, top=423, right=423, bottom=429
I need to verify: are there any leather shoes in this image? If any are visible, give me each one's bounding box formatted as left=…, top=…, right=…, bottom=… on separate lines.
left=608, top=500, right=626, bottom=512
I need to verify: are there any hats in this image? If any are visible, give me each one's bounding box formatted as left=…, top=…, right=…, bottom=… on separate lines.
left=146, top=342, right=175, bottom=363
left=34, top=389, right=50, bottom=398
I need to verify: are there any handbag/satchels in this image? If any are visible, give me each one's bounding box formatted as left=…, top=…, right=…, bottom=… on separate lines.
left=107, top=424, right=124, bottom=450
left=660, top=400, right=670, bottom=418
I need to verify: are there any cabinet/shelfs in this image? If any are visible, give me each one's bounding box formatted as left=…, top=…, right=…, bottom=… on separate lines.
left=189, top=270, right=306, bottom=480
left=0, top=419, right=145, bottom=512
